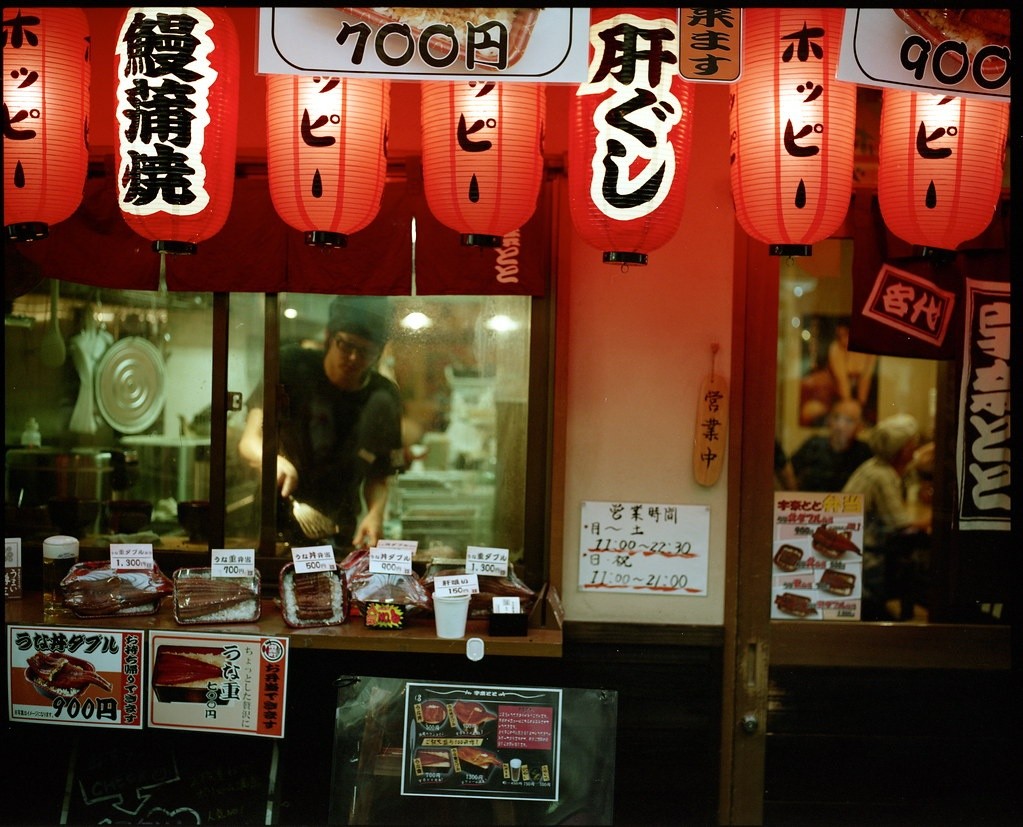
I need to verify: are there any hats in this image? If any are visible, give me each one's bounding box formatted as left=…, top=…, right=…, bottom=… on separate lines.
left=870, top=414, right=918, bottom=458
left=328, top=293, right=395, bottom=351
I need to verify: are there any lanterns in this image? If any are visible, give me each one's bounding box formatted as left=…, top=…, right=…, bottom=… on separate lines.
left=106, top=1, right=242, bottom=260
left=723, top=4, right=859, bottom=267
left=2, top=3, right=95, bottom=245
left=418, top=79, right=547, bottom=255
left=261, top=74, right=391, bottom=251
left=876, top=86, right=1010, bottom=266
left=562, top=8, right=695, bottom=272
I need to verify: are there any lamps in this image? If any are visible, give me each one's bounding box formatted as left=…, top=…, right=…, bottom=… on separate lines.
left=566, top=4, right=704, bottom=275
left=725, top=9, right=860, bottom=261
left=1, top=5, right=91, bottom=242
left=262, top=74, right=390, bottom=252
left=877, top=85, right=1011, bottom=262
left=417, top=81, right=549, bottom=249
left=111, top=7, right=243, bottom=255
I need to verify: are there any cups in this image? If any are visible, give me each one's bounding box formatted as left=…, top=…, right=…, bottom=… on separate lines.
left=42, top=536, right=80, bottom=618
left=432, top=590, right=472, bottom=638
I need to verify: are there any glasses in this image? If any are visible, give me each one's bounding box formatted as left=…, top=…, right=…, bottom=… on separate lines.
left=332, top=333, right=380, bottom=358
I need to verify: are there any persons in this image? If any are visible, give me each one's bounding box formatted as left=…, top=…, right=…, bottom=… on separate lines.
left=837, top=413, right=933, bottom=624
left=235, top=294, right=411, bottom=563
left=787, top=394, right=877, bottom=496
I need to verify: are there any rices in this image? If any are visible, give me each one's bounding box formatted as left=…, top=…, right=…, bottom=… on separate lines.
left=154, top=650, right=230, bottom=691
left=26, top=653, right=89, bottom=698
left=282, top=568, right=344, bottom=625
left=175, top=569, right=258, bottom=621
